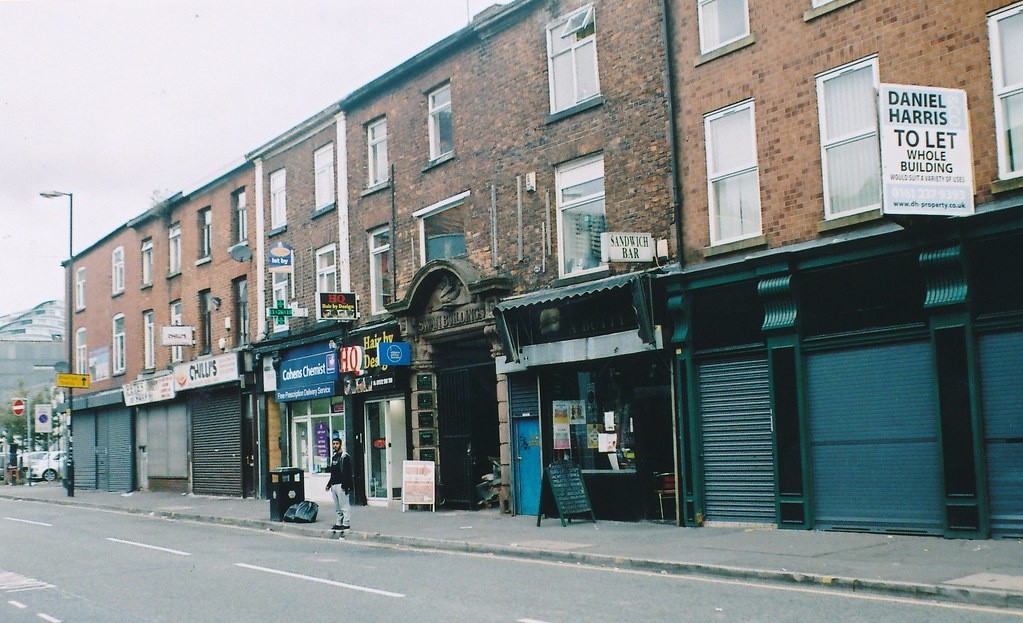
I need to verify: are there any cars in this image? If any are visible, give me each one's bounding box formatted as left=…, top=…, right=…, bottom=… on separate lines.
left=22, top=449, right=68, bottom=482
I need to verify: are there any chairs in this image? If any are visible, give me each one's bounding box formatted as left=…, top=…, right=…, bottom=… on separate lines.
left=655, top=474, right=687, bottom=521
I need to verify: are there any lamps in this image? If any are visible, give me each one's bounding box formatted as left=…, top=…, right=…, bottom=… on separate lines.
left=211, top=297, right=223, bottom=308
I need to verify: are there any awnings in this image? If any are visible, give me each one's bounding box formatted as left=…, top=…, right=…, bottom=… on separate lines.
left=492, top=273, right=658, bottom=364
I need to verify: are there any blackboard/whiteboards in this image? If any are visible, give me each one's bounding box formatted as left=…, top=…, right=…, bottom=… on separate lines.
left=538, top=460, right=592, bottom=515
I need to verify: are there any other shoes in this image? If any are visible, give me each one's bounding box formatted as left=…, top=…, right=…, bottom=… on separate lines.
left=328, top=525, right=350, bottom=532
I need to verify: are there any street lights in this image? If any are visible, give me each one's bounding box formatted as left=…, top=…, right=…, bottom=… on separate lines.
left=41, top=190, right=78, bottom=496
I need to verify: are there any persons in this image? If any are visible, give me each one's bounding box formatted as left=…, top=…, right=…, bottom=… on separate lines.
left=326, top=438, right=352, bottom=531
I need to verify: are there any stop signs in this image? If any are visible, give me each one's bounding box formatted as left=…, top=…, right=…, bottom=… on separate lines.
left=13, top=401, right=26, bottom=417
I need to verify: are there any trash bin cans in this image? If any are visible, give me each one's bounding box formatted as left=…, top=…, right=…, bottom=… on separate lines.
left=6, top=466, right=29, bottom=485
left=268, top=466, right=305, bottom=522
